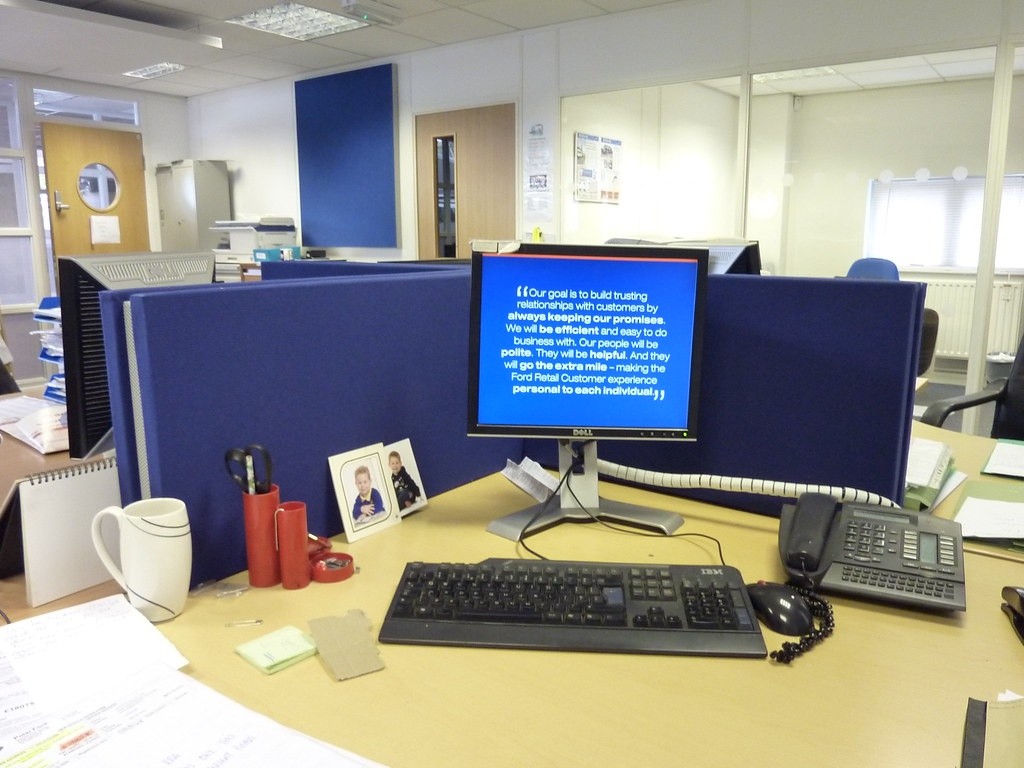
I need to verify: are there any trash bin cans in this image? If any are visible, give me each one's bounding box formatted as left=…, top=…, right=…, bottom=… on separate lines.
left=985, top=351, right=1016, bottom=382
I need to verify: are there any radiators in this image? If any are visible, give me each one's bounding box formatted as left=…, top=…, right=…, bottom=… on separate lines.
left=901, top=279, right=1024, bottom=362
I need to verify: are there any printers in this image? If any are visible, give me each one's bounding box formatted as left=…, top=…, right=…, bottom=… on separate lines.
left=209, top=214, right=298, bottom=283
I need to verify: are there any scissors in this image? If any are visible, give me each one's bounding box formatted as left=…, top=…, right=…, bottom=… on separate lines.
left=224, top=445, right=272, bottom=494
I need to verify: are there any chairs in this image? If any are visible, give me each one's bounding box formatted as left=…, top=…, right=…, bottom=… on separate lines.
left=921, top=333, right=1024, bottom=441
left=846, top=258, right=899, bottom=281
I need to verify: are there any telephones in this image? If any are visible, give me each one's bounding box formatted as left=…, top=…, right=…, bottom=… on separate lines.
left=778, top=492, right=966, bottom=615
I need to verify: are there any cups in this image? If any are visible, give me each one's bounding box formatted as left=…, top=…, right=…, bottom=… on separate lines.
left=91, top=497, right=192, bottom=622
left=276, top=500, right=311, bottom=590
left=242, top=483, right=279, bottom=586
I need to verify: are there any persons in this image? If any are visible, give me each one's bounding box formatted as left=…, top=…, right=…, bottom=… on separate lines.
left=388, top=451, right=423, bottom=512
left=352, top=467, right=383, bottom=520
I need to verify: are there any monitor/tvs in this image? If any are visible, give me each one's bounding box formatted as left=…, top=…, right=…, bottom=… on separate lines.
left=57, top=248, right=220, bottom=461
left=466, top=239, right=709, bottom=541
left=653, top=239, right=762, bottom=277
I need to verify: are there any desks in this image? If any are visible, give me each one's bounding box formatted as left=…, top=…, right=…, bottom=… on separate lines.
left=0, top=421, right=1024, bottom=768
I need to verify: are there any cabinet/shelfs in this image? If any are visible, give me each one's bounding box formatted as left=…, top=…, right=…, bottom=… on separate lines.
left=155, top=161, right=232, bottom=253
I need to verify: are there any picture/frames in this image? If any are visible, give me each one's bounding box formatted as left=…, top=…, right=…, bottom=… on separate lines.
left=385, top=438, right=429, bottom=517
left=327, top=443, right=403, bottom=545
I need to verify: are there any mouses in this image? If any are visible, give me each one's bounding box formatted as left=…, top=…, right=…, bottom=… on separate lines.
left=746, top=580, right=813, bottom=637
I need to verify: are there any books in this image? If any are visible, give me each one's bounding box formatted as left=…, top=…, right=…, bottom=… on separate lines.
left=905, top=437, right=944, bottom=489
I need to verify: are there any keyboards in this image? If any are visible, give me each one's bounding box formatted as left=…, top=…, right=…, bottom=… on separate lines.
left=378, top=557, right=768, bottom=659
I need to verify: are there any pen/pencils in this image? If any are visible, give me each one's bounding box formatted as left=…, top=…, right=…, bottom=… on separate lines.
left=245, top=455, right=256, bottom=495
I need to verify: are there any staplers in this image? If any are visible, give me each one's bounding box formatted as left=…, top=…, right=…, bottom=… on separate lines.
left=1001, top=586, right=1024, bottom=646
